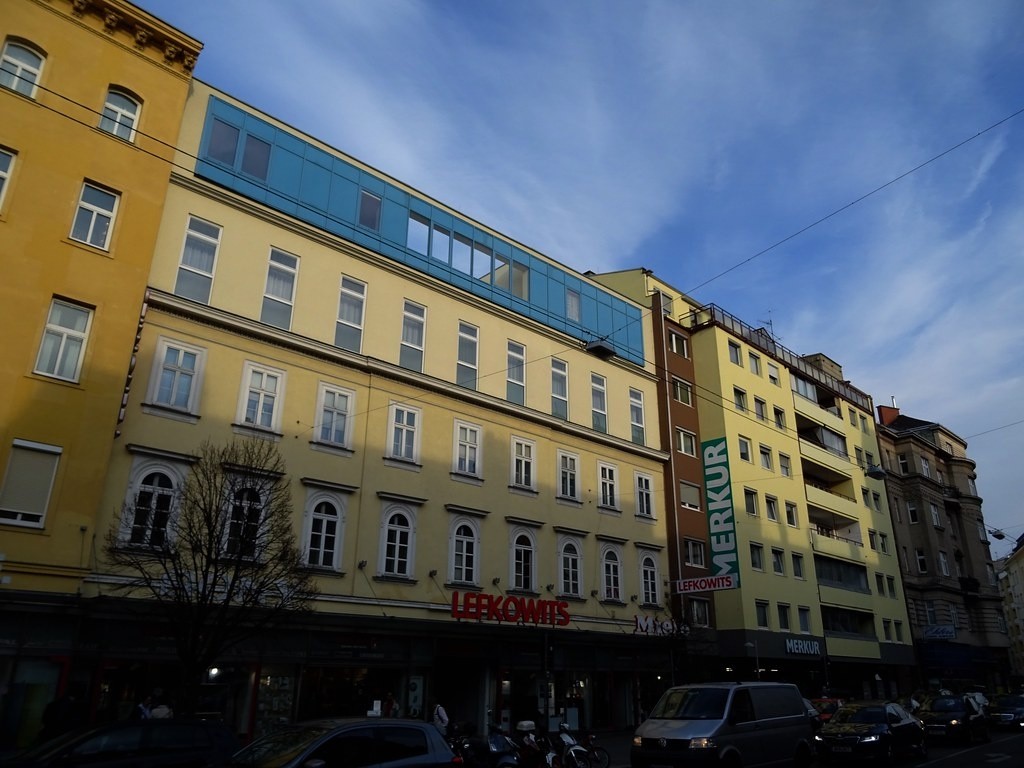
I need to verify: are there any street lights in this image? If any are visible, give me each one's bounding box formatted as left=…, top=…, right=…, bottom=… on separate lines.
left=744, top=642, right=761, bottom=682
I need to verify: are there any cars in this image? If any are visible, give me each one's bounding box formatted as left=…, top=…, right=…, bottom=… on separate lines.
left=912, top=693, right=992, bottom=748
left=813, top=703, right=927, bottom=768
left=990, top=695, right=1024, bottom=733
left=207, top=718, right=462, bottom=768
left=0, top=718, right=241, bottom=768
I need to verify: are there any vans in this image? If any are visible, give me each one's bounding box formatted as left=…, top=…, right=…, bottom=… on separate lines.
left=630, top=683, right=814, bottom=768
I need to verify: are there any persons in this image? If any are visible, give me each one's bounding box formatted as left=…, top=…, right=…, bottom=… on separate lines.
left=137, top=683, right=178, bottom=724
left=428, top=696, right=449, bottom=740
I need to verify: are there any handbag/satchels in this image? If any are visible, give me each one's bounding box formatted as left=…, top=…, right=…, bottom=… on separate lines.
left=438, top=707, right=461, bottom=736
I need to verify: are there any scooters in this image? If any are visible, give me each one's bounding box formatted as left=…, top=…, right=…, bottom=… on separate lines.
left=448, top=708, right=611, bottom=768
